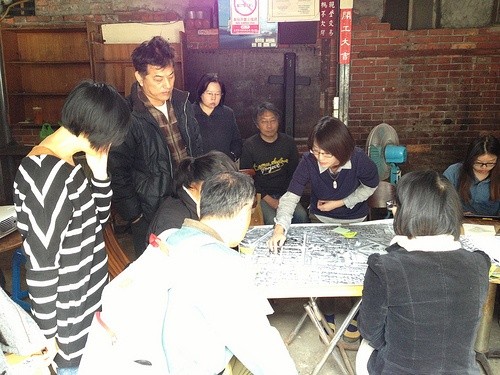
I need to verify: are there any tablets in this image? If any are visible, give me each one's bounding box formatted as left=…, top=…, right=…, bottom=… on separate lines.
left=463, top=215, right=500, bottom=219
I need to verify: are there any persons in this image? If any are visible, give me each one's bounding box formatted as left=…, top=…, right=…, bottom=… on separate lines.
left=11, top=79, right=133, bottom=375
left=355, top=168, right=492, bottom=375
left=145, top=151, right=239, bottom=251
left=442, top=134, right=500, bottom=215
left=106, top=36, right=204, bottom=259
left=240, top=100, right=309, bottom=225
left=101, top=171, right=299, bottom=375
left=188, top=73, right=244, bottom=162
left=266, top=116, right=380, bottom=344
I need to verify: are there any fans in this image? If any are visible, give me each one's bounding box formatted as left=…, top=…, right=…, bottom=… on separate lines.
left=365, top=123, right=407, bottom=218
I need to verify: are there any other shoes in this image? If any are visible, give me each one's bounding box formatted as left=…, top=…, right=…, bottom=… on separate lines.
left=319, top=319, right=336, bottom=344
left=343, top=320, right=361, bottom=343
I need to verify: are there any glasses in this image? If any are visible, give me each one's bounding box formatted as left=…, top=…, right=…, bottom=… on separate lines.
left=473, top=161, right=497, bottom=168
left=386, top=200, right=397, bottom=212
left=204, top=92, right=223, bottom=97
left=310, top=149, right=334, bottom=158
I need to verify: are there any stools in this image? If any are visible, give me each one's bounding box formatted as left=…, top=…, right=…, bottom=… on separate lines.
left=12, top=248, right=30, bottom=311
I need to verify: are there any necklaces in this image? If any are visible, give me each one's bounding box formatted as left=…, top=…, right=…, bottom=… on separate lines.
left=326, top=167, right=342, bottom=189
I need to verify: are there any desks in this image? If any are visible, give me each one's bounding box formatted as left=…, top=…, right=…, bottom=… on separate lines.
left=238, top=218, right=500, bottom=375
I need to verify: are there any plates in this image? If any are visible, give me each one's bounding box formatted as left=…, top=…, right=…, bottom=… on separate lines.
left=19, top=121, right=59, bottom=128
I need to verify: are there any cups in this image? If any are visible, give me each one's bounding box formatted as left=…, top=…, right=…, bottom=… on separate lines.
left=32, top=107, right=42, bottom=123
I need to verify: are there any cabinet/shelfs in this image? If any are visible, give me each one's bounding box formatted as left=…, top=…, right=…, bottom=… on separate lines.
left=0, top=21, right=94, bottom=128
left=92, top=31, right=188, bottom=93
